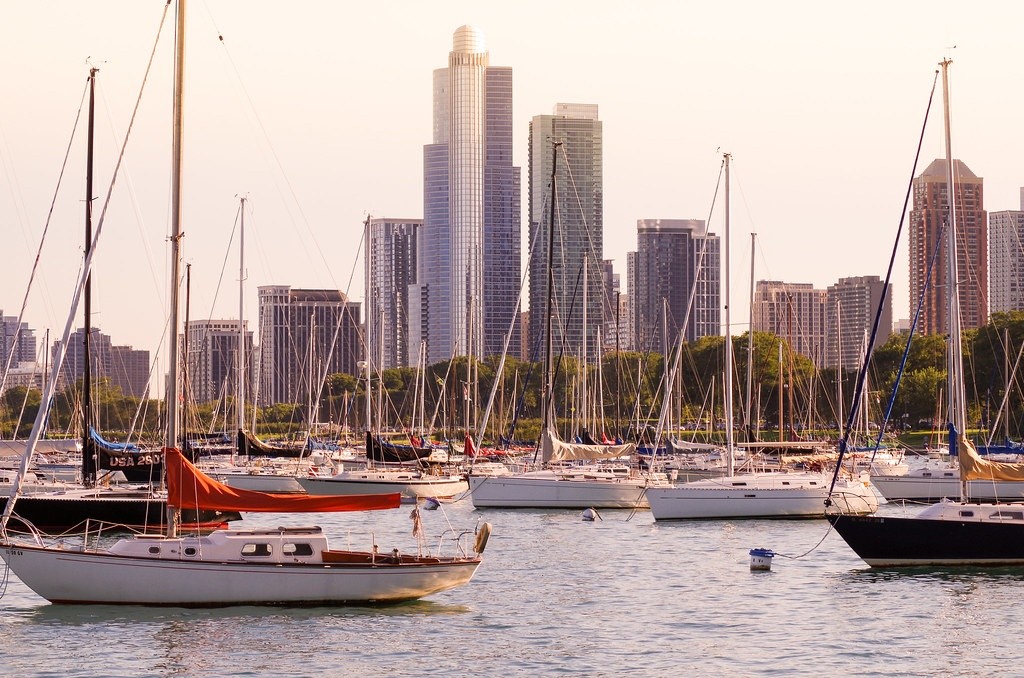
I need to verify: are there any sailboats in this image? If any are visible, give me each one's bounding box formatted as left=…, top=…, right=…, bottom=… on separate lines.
left=0, top=0, right=828, bottom=607
left=870, top=207, right=1024, bottom=503
left=824, top=57, right=1024, bottom=568
left=645, top=154, right=879, bottom=520
